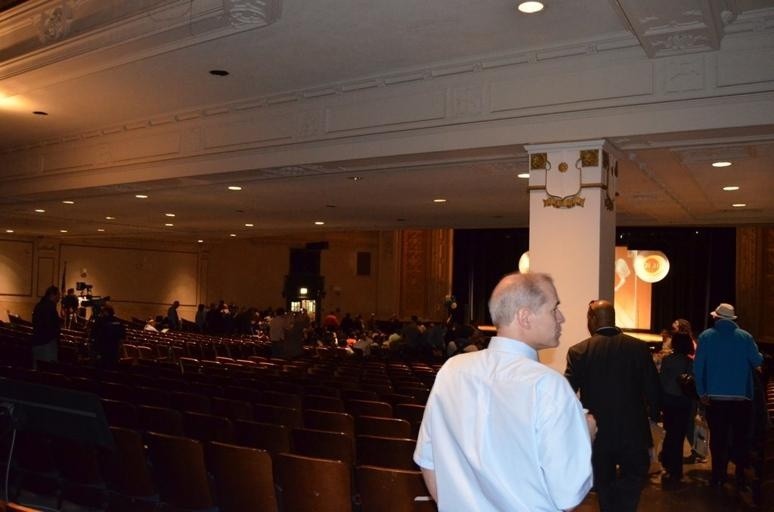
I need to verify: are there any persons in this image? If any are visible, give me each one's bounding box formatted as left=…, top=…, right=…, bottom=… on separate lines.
left=413, top=272, right=599, bottom=512
left=693, top=303, right=765, bottom=487
left=563, top=298, right=663, bottom=512
left=661, top=329, right=672, bottom=353
left=671, top=319, right=706, bottom=464
left=659, top=332, right=699, bottom=482
left=31, top=287, right=128, bottom=369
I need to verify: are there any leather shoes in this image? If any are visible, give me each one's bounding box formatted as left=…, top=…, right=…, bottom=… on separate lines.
left=658, top=451, right=747, bottom=491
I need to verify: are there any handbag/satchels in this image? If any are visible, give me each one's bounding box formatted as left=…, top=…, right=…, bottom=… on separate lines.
left=691, top=414, right=709, bottom=460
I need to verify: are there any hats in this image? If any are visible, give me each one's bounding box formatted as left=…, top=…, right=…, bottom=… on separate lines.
left=709, top=302, right=738, bottom=320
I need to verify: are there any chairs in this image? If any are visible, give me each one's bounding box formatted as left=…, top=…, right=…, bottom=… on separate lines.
left=762, top=375, right=774, bottom=512
left=1, top=310, right=445, bottom=510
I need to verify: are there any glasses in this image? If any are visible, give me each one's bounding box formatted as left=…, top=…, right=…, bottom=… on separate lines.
left=589, top=300, right=600, bottom=322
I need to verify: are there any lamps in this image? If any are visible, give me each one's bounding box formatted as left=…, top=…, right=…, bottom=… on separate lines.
left=515, top=241, right=671, bottom=291
